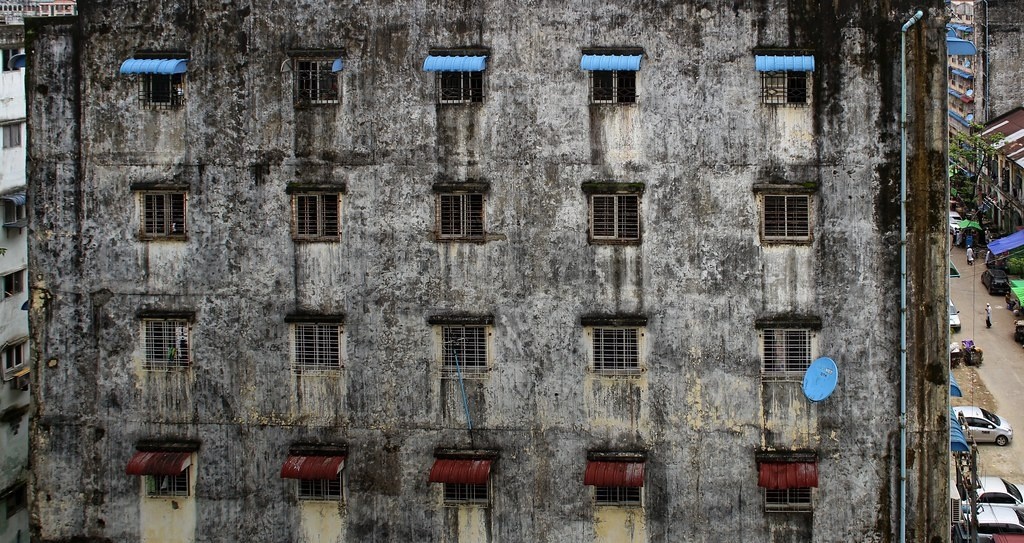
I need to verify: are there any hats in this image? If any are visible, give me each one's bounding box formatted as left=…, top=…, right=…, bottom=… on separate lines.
left=1007, top=291, right=1009, bottom=293
left=968, top=245, right=970, bottom=247
left=986, top=303, right=990, bottom=305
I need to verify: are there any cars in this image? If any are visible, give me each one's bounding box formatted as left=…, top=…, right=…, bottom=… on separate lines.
left=963, top=505, right=1024, bottom=543
left=949, top=298, right=961, bottom=331
left=962, top=476, right=1024, bottom=514
left=952, top=405, right=1013, bottom=446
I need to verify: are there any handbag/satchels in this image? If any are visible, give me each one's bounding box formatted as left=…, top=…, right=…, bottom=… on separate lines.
left=987, top=316, right=991, bottom=327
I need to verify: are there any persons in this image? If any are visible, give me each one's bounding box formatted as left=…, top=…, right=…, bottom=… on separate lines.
left=985, top=303, right=992, bottom=329
left=1006, top=291, right=1015, bottom=311
left=966, top=244, right=974, bottom=265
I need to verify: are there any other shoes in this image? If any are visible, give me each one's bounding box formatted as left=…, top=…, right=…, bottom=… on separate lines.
left=986, top=327, right=990, bottom=328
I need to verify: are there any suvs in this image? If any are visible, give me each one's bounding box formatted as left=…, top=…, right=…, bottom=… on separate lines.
left=981, top=269, right=1011, bottom=294
left=949, top=212, right=963, bottom=233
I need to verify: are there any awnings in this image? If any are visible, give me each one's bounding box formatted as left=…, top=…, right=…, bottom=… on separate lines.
left=281, top=456, right=345, bottom=479
left=950, top=371, right=962, bottom=398
left=580, top=52, right=642, bottom=70
left=958, top=219, right=981, bottom=230
left=423, top=54, right=487, bottom=71
left=430, top=459, right=491, bottom=484
left=1010, top=279, right=1024, bottom=307
left=583, top=461, right=645, bottom=487
left=125, top=451, right=192, bottom=476
left=119, top=58, right=187, bottom=74
left=759, top=461, right=818, bottom=489
left=987, top=229, right=1024, bottom=254
left=950, top=405, right=970, bottom=451
left=755, top=54, right=815, bottom=72
